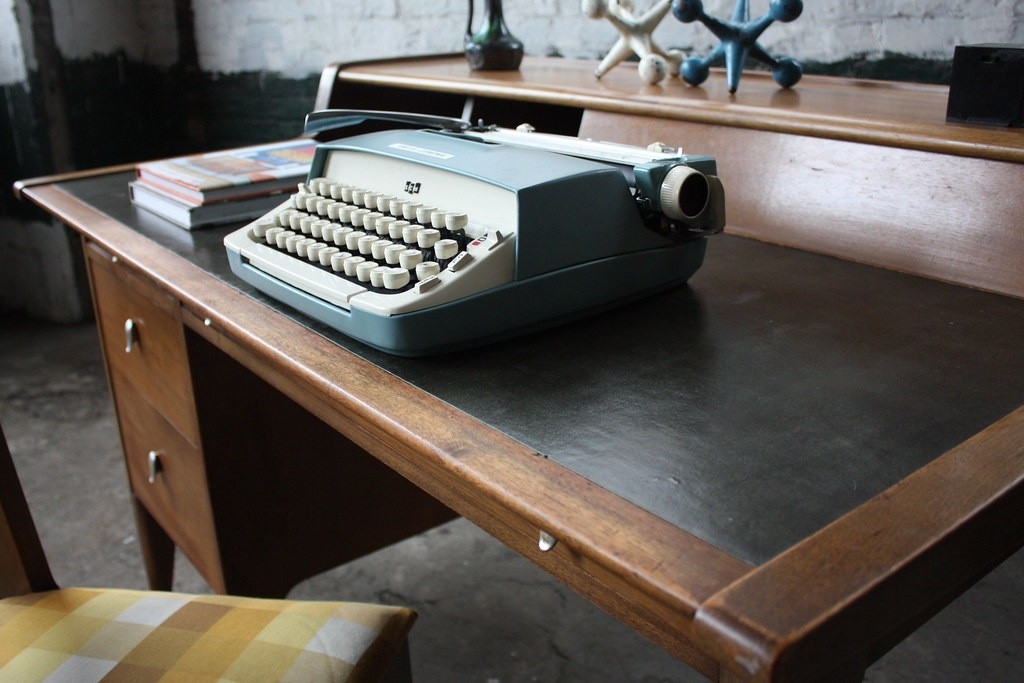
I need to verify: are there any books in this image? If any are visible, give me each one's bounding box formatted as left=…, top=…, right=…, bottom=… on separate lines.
left=128, top=136, right=320, bottom=232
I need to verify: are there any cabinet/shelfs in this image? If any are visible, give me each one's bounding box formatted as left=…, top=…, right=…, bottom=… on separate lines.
left=14, top=51, right=1024, bottom=682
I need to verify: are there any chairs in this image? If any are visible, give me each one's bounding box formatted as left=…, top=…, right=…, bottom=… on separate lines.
left=0, top=425, right=418, bottom=683
